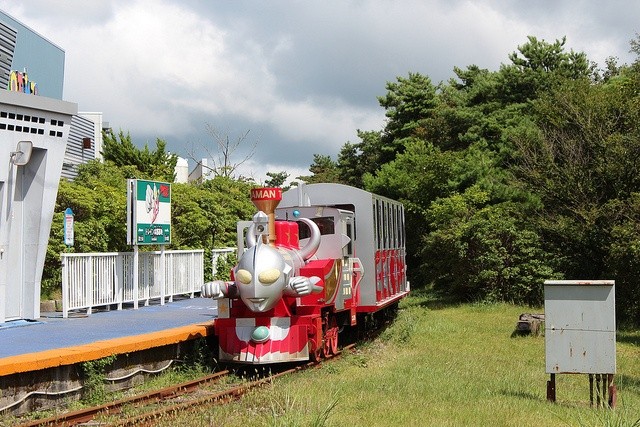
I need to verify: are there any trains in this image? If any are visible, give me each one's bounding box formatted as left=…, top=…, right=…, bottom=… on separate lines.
left=201, top=181, right=410, bottom=363
